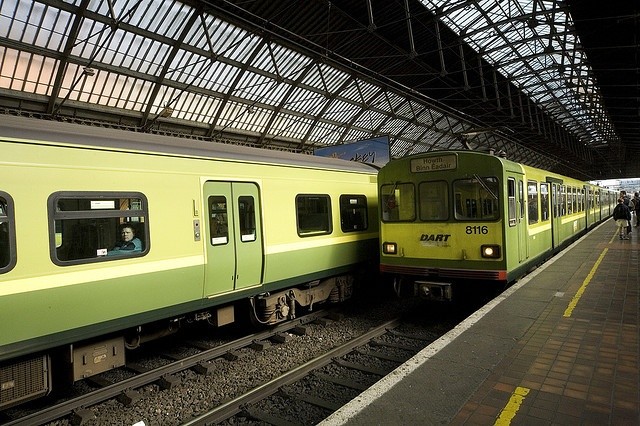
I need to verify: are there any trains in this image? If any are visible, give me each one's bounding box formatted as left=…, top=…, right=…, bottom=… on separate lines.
left=0, top=111, right=376, bottom=412
left=377, top=126, right=634, bottom=306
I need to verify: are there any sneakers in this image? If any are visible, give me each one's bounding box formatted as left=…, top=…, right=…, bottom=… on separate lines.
left=623, top=236, right=629, bottom=240
left=619, top=236, right=622, bottom=240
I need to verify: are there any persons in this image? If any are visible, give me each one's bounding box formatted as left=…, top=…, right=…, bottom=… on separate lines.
left=613, top=198, right=631, bottom=240
left=620, top=191, right=632, bottom=235
left=631, top=193, right=640, bottom=227
left=105, top=223, right=142, bottom=255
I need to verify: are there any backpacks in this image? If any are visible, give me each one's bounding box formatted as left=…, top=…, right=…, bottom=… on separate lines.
left=629, top=200, right=635, bottom=212
